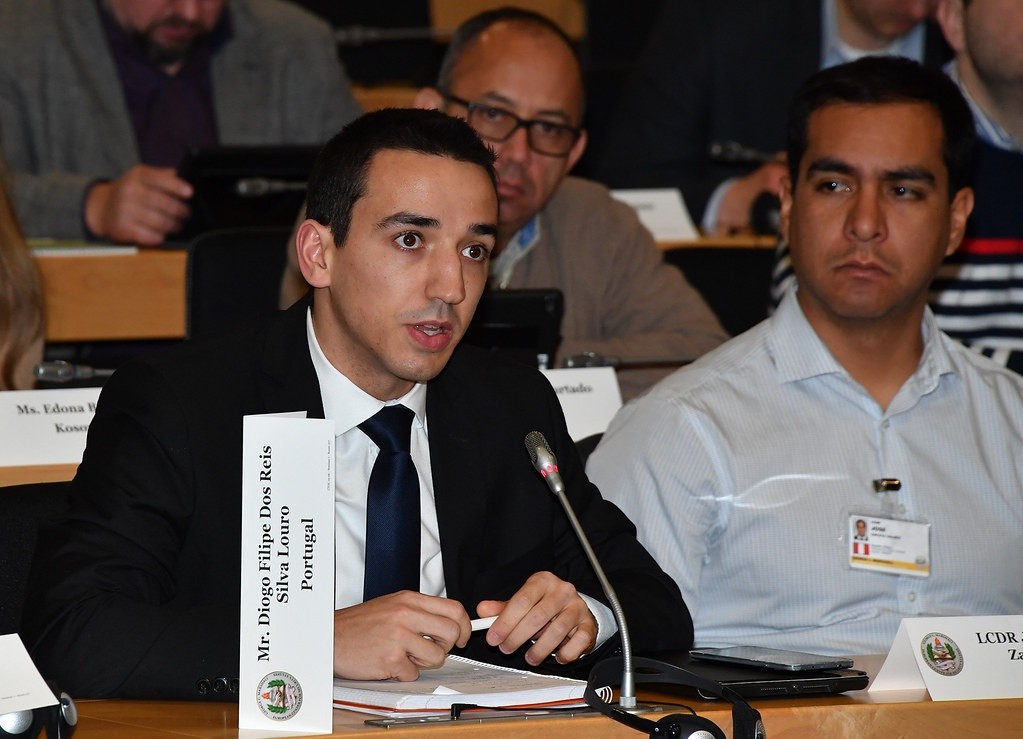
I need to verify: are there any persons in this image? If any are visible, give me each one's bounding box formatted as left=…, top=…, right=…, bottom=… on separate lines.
left=20, top=108, right=695, bottom=700
left=585, top=56, right=1023, bottom=657
left=0, top=0, right=1023, bottom=410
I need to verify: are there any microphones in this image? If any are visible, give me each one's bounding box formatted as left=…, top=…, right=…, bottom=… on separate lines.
left=524, top=430, right=636, bottom=708
left=34, top=361, right=115, bottom=382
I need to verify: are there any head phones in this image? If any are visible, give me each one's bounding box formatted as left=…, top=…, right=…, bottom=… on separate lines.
left=583, top=656, right=766, bottom=739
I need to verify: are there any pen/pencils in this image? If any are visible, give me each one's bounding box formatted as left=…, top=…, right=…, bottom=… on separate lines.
left=423, top=614, right=501, bottom=640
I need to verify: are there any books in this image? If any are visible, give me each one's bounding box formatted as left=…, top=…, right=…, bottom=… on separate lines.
left=331, top=652, right=590, bottom=719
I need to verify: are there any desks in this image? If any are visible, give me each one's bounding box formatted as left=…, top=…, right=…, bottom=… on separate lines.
left=0, top=88, right=1022, bottom=739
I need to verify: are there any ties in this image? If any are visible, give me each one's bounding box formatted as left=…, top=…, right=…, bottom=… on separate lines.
left=356, top=403, right=422, bottom=603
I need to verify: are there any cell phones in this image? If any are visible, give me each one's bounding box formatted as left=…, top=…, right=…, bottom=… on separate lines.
left=689, top=646, right=855, bottom=671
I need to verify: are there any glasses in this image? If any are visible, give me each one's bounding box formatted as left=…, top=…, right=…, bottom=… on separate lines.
left=442, top=91, right=581, bottom=158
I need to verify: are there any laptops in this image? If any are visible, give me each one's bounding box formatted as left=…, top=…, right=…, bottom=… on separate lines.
left=162, top=143, right=325, bottom=246
left=551, top=655, right=870, bottom=702
